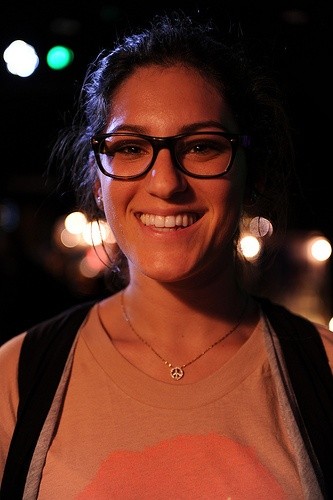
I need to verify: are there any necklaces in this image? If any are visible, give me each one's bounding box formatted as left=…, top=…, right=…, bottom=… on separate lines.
left=121, top=287, right=248, bottom=382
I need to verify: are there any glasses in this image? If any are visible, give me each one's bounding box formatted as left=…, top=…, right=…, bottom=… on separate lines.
left=83, top=132, right=236, bottom=179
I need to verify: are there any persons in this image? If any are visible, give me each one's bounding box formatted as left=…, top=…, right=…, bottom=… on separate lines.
left=0, top=25, right=333, bottom=500
